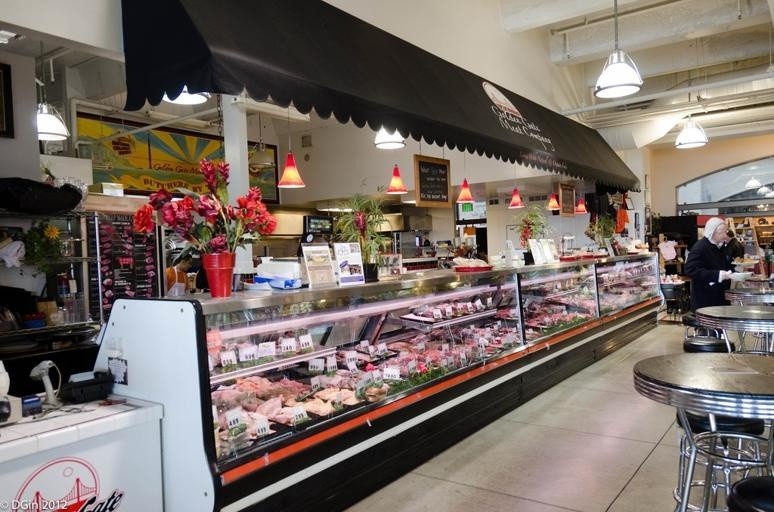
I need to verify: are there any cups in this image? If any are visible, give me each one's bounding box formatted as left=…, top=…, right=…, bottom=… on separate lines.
left=23, top=272, right=86, bottom=328
left=650, top=233, right=684, bottom=245
left=736, top=217, right=774, bottom=246
left=175, top=283, right=209, bottom=296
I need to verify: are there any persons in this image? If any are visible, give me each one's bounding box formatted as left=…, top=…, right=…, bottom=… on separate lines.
left=194, top=267, right=208, bottom=293
left=163, top=253, right=193, bottom=297
left=725, top=229, right=744, bottom=260
left=682, top=214, right=737, bottom=338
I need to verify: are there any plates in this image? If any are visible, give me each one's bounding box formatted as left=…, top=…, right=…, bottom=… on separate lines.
left=732, top=260, right=760, bottom=265
left=559, top=252, right=639, bottom=262
left=456, top=266, right=493, bottom=271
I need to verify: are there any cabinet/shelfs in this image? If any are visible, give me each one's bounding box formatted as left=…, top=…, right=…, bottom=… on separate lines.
left=729, top=219, right=765, bottom=277
left=0, top=213, right=103, bottom=339
left=755, top=225, right=774, bottom=246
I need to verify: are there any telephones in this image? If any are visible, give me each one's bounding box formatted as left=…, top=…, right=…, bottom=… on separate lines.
left=56, top=372, right=115, bottom=404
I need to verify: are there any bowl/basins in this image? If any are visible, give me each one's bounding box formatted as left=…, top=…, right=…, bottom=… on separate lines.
left=418, top=237, right=463, bottom=257
left=733, top=273, right=751, bottom=279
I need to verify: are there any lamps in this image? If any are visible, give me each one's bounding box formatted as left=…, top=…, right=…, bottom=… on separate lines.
left=675, top=71, right=709, bottom=148
left=745, top=167, right=774, bottom=199
left=594, top=0, right=644, bottom=98
left=249, top=111, right=275, bottom=171
left=162, top=85, right=210, bottom=108
left=35, top=43, right=69, bottom=142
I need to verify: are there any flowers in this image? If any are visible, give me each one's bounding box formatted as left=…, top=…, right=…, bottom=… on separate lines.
left=21, top=222, right=65, bottom=277
left=327, top=187, right=401, bottom=262
left=133, top=160, right=277, bottom=253
left=511, top=205, right=548, bottom=252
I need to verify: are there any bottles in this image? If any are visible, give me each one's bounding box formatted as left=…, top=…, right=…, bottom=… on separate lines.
left=233, top=272, right=257, bottom=290
left=60, top=230, right=74, bottom=256
left=661, top=273, right=679, bottom=283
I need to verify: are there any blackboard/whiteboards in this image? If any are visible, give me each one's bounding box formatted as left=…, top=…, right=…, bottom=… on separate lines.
left=559, top=183, right=576, bottom=217
left=414, top=155, right=453, bottom=209
